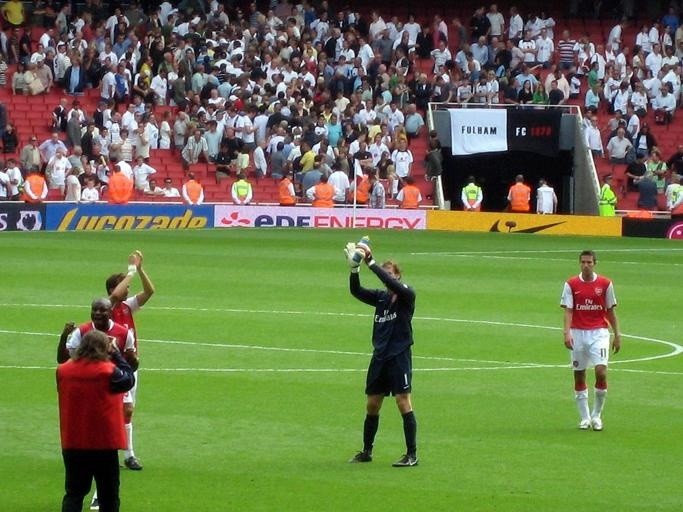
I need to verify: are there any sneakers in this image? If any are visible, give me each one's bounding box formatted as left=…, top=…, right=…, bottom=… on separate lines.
left=124, top=456, right=142, bottom=470
left=89, top=492, right=99, bottom=510
left=392, top=455, right=419, bottom=466
left=348, top=452, right=372, bottom=463
left=579, top=417, right=602, bottom=430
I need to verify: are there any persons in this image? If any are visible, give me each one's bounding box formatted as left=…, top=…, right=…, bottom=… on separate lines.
left=54, top=330, right=134, bottom=512
left=462, top=177, right=483, bottom=212
left=507, top=174, right=533, bottom=214
left=558, top=249, right=621, bottom=433
left=536, top=180, right=557, bottom=215
left=427, top=1, right=595, bottom=108
left=0, top=1, right=206, bottom=206
left=57, top=298, right=139, bottom=510
left=200, top=4, right=432, bottom=209
left=104, top=250, right=154, bottom=469
left=581, top=5, right=682, bottom=216
left=343, top=234, right=418, bottom=468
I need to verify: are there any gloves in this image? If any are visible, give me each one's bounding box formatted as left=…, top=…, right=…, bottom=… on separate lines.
left=344, top=242, right=373, bottom=268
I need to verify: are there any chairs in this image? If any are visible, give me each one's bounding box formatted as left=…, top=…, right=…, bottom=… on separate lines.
left=0, top=6, right=683, bottom=218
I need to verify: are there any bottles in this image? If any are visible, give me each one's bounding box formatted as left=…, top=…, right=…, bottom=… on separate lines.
left=351, top=235, right=370, bottom=263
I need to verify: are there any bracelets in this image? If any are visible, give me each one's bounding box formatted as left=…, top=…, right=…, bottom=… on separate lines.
left=127, top=264, right=136, bottom=276
left=613, top=329, right=620, bottom=336
